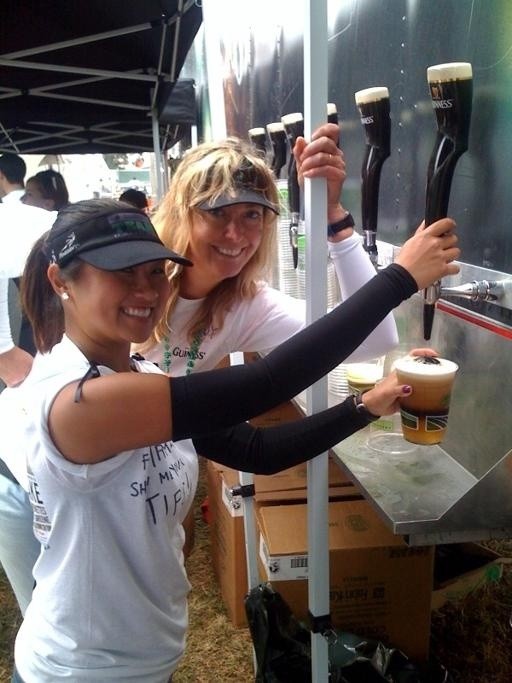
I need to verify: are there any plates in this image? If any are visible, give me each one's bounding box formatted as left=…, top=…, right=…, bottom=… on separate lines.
left=329, top=363, right=349, bottom=397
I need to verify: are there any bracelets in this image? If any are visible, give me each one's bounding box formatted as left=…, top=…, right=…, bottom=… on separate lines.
left=328, top=210, right=354, bottom=237
left=355, top=386, right=380, bottom=423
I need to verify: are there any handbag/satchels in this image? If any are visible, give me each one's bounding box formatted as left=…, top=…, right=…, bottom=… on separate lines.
left=244, top=584, right=450, bottom=683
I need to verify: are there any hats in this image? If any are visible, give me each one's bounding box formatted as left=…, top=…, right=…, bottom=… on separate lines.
left=191, top=187, right=280, bottom=218
left=44, top=208, right=195, bottom=272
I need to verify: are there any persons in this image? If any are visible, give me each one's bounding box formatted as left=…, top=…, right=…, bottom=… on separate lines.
left=0, top=150, right=152, bottom=390
left=0, top=121, right=398, bottom=620
left=9, top=196, right=461, bottom=683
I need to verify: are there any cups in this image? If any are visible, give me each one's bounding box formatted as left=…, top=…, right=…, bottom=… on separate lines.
left=275, top=213, right=339, bottom=311
left=394, top=356, right=459, bottom=445
left=346, top=362, right=379, bottom=394
